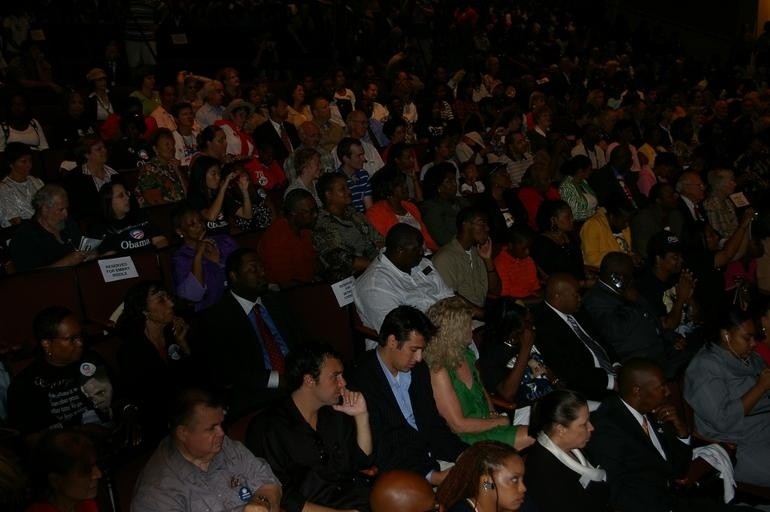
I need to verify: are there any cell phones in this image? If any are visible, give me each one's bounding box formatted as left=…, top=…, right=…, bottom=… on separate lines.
left=608, top=271, right=625, bottom=290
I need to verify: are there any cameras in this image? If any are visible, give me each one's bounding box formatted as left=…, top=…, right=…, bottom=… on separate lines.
left=749, top=209, right=758, bottom=221
left=130, top=110, right=146, bottom=133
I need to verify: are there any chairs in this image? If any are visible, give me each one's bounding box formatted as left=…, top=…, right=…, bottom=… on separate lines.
left=288, top=272, right=357, bottom=353
left=0, top=267, right=80, bottom=364
left=87, top=249, right=164, bottom=315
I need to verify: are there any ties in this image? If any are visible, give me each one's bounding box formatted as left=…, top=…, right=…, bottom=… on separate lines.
left=568, top=315, right=612, bottom=373
left=643, top=415, right=651, bottom=435
left=250, top=303, right=285, bottom=375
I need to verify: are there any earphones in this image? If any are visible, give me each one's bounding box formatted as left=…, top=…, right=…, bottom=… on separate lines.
left=725, top=335, right=728, bottom=342
left=483, top=481, right=494, bottom=490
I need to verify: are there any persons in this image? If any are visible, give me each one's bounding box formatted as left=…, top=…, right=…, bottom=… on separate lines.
left=1, top=2, right=769, bottom=512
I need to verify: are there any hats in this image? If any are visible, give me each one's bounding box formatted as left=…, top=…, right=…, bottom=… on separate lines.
left=86, top=68, right=106, bottom=82
left=486, top=161, right=509, bottom=178
left=221, top=98, right=255, bottom=122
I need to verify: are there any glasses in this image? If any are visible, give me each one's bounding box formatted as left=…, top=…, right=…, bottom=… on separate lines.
left=684, top=182, right=704, bottom=189
left=353, top=120, right=369, bottom=126
